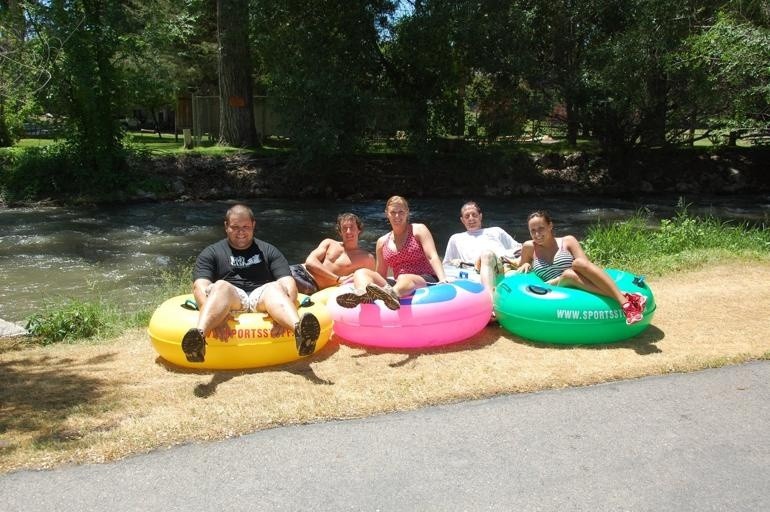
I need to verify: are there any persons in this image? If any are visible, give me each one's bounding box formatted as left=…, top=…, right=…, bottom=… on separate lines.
left=516, top=209, right=649, bottom=326
left=181, top=203, right=321, bottom=363
left=442, top=200, right=523, bottom=323
left=335, top=195, right=447, bottom=312
left=287, top=211, right=376, bottom=295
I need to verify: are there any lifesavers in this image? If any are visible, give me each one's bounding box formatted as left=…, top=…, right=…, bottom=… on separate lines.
left=495, top=269, right=655, bottom=345
left=147, top=293, right=335, bottom=370
left=326, top=276, right=492, bottom=348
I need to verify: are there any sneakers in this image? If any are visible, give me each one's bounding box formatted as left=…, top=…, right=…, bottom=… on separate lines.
left=335, top=292, right=375, bottom=309
left=623, top=292, right=648, bottom=326
left=293, top=313, right=320, bottom=356
left=182, top=328, right=207, bottom=364
left=365, top=282, right=401, bottom=311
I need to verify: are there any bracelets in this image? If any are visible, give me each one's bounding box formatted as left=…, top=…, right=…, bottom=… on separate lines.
left=336, top=276, right=341, bottom=283
